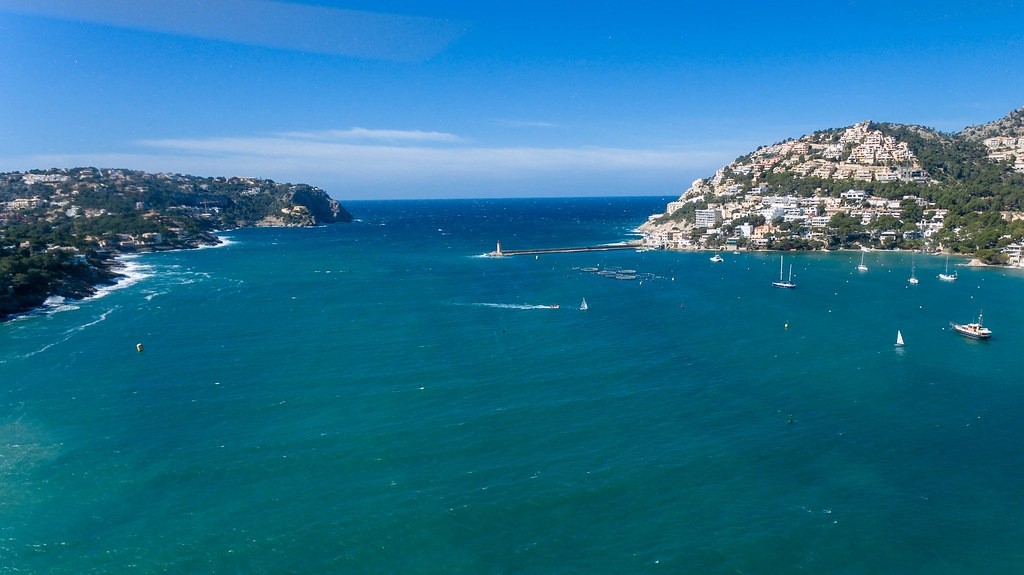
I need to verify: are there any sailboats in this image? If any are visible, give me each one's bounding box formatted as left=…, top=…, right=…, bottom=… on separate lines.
left=894, top=329, right=907, bottom=347
left=579, top=297, right=589, bottom=310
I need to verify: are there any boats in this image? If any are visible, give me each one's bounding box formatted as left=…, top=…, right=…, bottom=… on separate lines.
left=909, top=276, right=919, bottom=284
left=580, top=266, right=638, bottom=280
left=772, top=255, right=796, bottom=288
left=949, top=307, right=993, bottom=340
left=936, top=253, right=958, bottom=281
left=857, top=251, right=868, bottom=272
left=709, top=255, right=725, bottom=263
